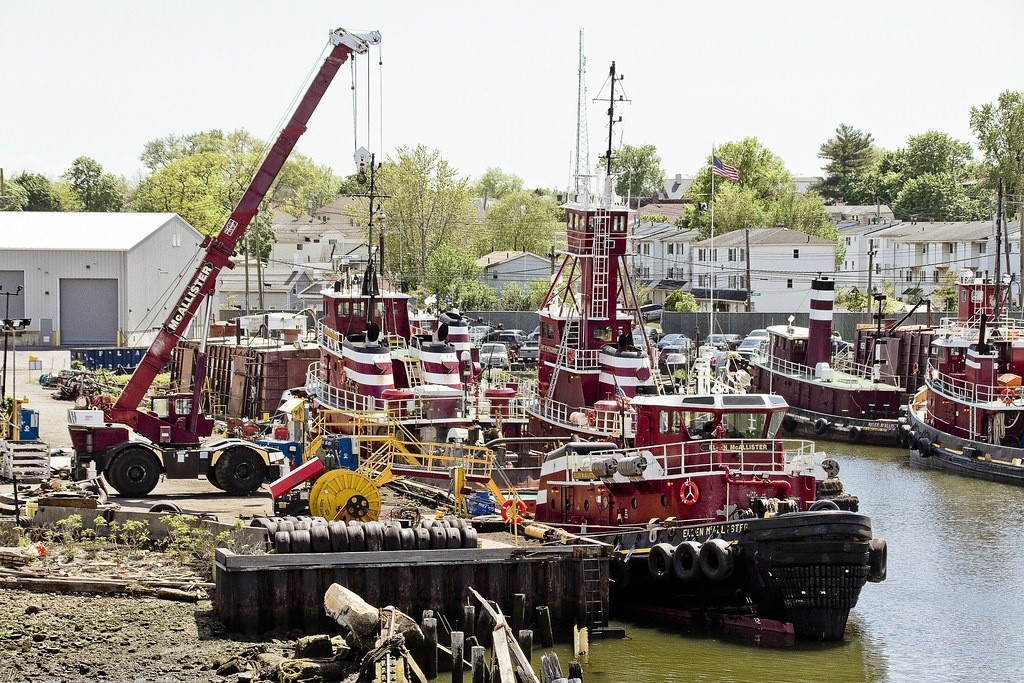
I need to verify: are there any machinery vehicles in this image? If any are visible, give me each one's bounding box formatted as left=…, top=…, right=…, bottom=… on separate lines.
left=66, top=25, right=383, bottom=497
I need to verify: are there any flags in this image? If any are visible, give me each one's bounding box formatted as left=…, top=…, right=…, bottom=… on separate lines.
left=713, top=155, right=740, bottom=181
left=614, top=384, right=632, bottom=410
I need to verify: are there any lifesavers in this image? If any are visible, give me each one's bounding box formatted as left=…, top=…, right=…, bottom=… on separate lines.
left=680, top=481, right=699, bottom=505
left=897, top=416, right=934, bottom=458
left=248, top=515, right=479, bottom=554
left=809, top=500, right=840, bottom=510
left=1000, top=389, right=1015, bottom=404
left=566, top=349, right=575, bottom=364
left=847, top=425, right=863, bottom=444
left=501, top=499, right=526, bottom=524
left=814, top=418, right=829, bottom=435
left=649, top=539, right=734, bottom=581
left=868, top=539, right=888, bottom=584
left=587, top=411, right=596, bottom=426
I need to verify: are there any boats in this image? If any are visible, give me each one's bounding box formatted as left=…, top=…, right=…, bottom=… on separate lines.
left=897, top=313, right=1024, bottom=483
left=742, top=271, right=911, bottom=445
left=271, top=58, right=887, bottom=647
left=854, top=177, right=1024, bottom=408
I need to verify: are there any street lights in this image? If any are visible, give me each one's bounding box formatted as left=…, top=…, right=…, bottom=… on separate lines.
left=0, top=285, right=24, bottom=399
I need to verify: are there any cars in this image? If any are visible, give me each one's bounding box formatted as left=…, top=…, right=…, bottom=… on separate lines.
left=631, top=304, right=664, bottom=323
left=657, top=334, right=692, bottom=351
left=468, top=323, right=541, bottom=368
left=712, top=352, right=742, bottom=371
left=832, top=337, right=849, bottom=355
left=745, top=328, right=769, bottom=340
left=724, top=333, right=744, bottom=350
left=658, top=346, right=693, bottom=374
left=736, top=336, right=770, bottom=362
left=670, top=338, right=695, bottom=351
left=704, top=333, right=725, bottom=349
left=632, top=327, right=666, bottom=344
left=831, top=329, right=842, bottom=342
